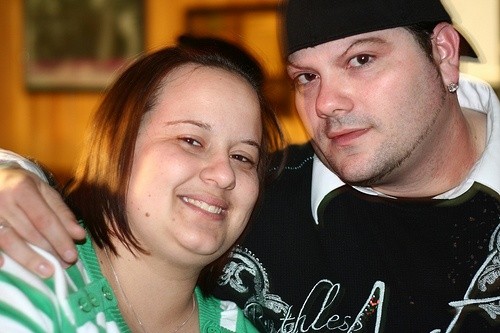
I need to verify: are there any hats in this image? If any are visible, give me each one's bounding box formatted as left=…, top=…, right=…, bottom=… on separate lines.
left=284, top=0, right=478, bottom=59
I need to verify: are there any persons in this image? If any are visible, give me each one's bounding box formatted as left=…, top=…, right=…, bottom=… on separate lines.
left=0, top=0, right=500, bottom=333
left=1, top=46, right=262, bottom=333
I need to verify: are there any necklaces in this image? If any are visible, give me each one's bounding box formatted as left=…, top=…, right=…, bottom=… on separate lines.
left=97, top=231, right=195, bottom=333
